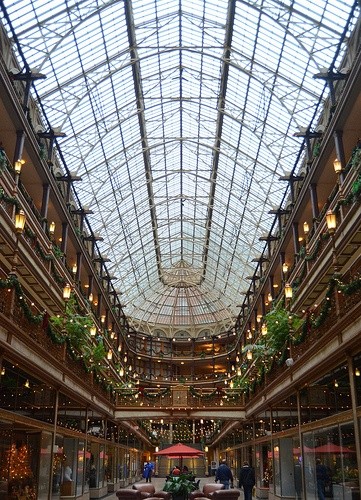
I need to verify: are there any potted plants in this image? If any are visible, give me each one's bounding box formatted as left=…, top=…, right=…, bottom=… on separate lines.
left=163, top=475, right=197, bottom=500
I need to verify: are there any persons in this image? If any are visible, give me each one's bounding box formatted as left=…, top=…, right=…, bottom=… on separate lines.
left=238, top=461, right=256, bottom=500
left=316, top=458, right=330, bottom=500
left=294, top=465, right=303, bottom=500
left=143, top=462, right=153, bottom=483
left=170, top=465, right=188, bottom=477
left=215, top=460, right=234, bottom=490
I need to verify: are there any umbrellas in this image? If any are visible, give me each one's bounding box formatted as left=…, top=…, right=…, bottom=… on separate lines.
left=40, top=447, right=109, bottom=468
left=255, top=443, right=355, bottom=470
left=153, top=442, right=205, bottom=468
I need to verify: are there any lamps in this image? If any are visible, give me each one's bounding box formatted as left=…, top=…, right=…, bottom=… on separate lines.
left=13, top=159, right=341, bottom=360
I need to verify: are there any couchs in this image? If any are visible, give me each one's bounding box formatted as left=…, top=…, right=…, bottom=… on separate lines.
left=116, top=484, right=170, bottom=500
left=190, top=484, right=240, bottom=500
